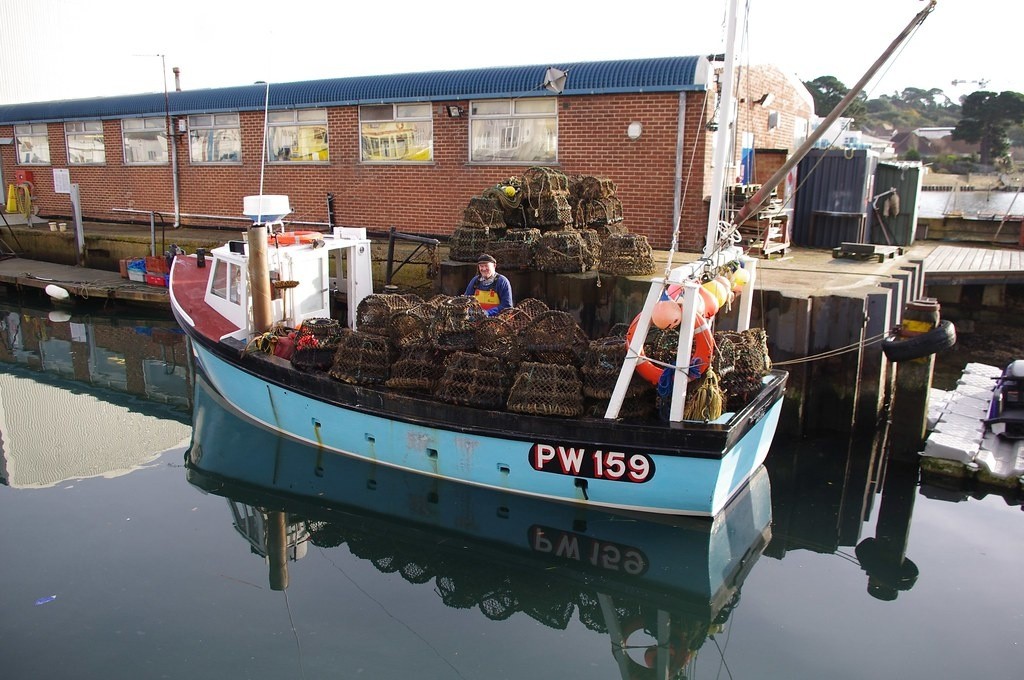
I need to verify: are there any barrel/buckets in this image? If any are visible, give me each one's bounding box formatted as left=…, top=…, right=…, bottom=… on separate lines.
left=48, top=221, right=58, bottom=232
left=59, top=223, right=67, bottom=232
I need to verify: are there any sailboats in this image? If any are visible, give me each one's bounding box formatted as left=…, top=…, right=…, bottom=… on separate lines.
left=183, top=363, right=772, bottom=680
left=169, top=0, right=789, bottom=519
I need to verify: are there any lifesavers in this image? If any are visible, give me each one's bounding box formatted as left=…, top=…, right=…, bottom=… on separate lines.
left=267, top=230, right=323, bottom=245
left=881, top=319, right=958, bottom=363
left=626, top=300, right=715, bottom=389
left=610, top=615, right=691, bottom=677
left=855, top=537, right=920, bottom=591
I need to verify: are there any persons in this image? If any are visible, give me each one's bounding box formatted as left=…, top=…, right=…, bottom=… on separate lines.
left=464, top=253, right=513, bottom=321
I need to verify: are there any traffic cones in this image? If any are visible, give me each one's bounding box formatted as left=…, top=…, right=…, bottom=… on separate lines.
left=5, top=184, right=19, bottom=214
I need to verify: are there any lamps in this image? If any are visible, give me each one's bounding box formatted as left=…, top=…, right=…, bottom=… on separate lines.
left=753, top=93, right=776, bottom=109
left=706, top=122, right=719, bottom=131
left=447, top=105, right=464, bottom=117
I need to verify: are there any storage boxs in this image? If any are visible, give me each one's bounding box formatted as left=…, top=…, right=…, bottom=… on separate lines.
left=119, top=255, right=170, bottom=287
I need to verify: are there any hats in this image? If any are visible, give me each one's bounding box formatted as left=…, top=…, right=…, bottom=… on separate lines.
left=477, top=254, right=496, bottom=264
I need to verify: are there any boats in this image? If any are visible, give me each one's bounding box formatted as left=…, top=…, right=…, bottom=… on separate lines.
left=289, top=121, right=431, bottom=162
left=34, top=136, right=106, bottom=164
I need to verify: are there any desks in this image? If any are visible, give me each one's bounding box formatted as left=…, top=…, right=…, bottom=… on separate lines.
left=807, top=209, right=868, bottom=247
